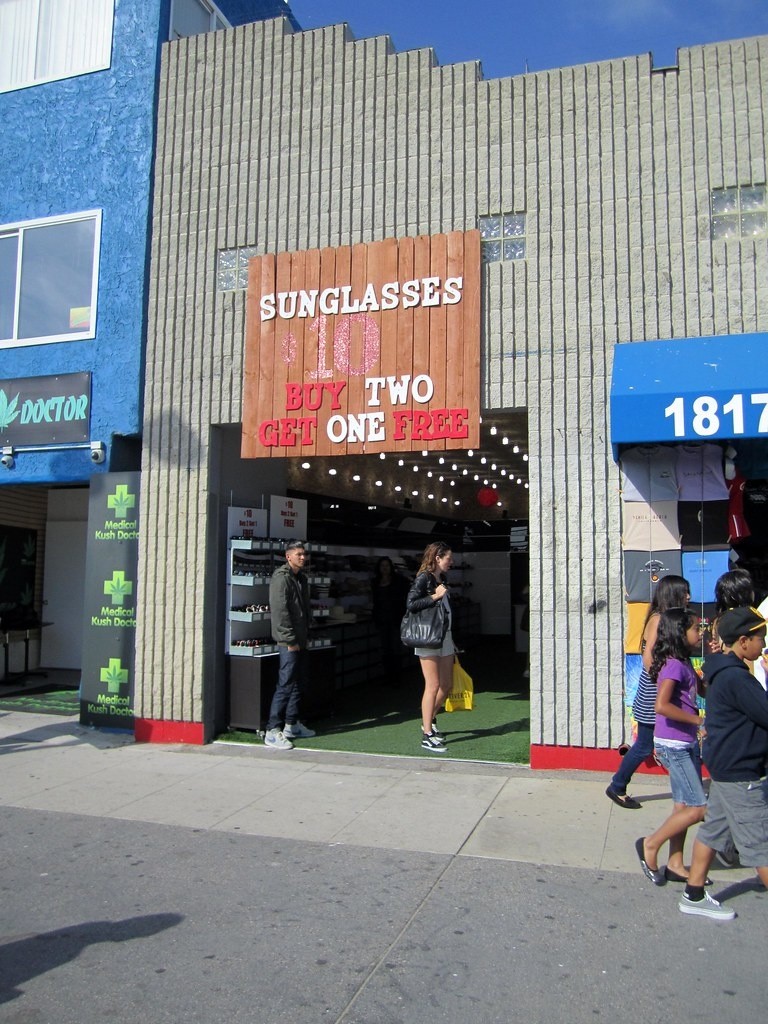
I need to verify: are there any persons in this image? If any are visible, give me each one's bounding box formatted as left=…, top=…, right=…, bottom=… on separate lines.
left=264, top=539, right=318, bottom=749
left=512, top=566, right=529, bottom=678
left=710, top=569, right=755, bottom=868
left=605, top=575, right=691, bottom=809
left=406, top=541, right=458, bottom=753
left=634, top=604, right=721, bottom=887
left=723, top=445, right=751, bottom=543
left=677, top=605, right=768, bottom=920
left=350, top=556, right=413, bottom=693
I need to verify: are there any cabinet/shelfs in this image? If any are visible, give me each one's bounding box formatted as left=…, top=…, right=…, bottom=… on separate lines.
left=225, top=536, right=337, bottom=735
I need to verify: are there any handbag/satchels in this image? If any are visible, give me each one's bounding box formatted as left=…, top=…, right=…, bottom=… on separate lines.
left=444, top=654, right=474, bottom=711
left=400, top=572, right=449, bottom=648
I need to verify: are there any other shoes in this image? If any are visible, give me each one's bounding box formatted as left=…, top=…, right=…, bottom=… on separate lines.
left=635, top=837, right=659, bottom=881
left=605, top=786, right=643, bottom=809
left=664, top=866, right=713, bottom=885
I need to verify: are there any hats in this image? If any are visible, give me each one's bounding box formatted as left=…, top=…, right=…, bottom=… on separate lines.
left=717, top=606, right=768, bottom=642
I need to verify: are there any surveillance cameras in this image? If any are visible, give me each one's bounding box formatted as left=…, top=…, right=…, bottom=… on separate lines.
left=91, top=450, right=105, bottom=464
left=2, top=456, right=14, bottom=467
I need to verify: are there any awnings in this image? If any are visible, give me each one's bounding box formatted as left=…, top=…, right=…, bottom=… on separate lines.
left=609, top=331, right=768, bottom=462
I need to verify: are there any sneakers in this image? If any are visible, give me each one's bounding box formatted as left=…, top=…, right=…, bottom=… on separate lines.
left=678, top=890, right=734, bottom=920
left=421, top=733, right=447, bottom=753
left=421, top=718, right=444, bottom=741
left=283, top=721, right=315, bottom=737
left=265, top=730, right=293, bottom=750
